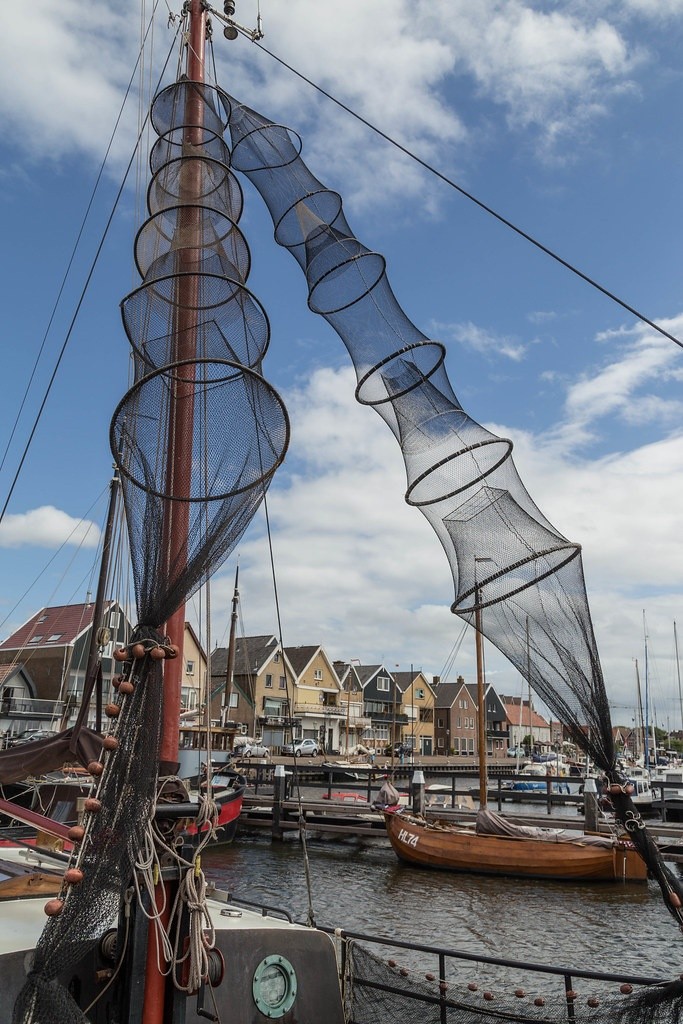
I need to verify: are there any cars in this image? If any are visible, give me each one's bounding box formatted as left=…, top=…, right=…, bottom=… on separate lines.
left=507, top=747, right=525, bottom=758
left=233, top=742, right=268, bottom=757
left=384, top=742, right=413, bottom=757
left=280, top=738, right=321, bottom=757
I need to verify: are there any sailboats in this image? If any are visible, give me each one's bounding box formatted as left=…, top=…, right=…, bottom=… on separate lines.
left=371, top=555, right=650, bottom=881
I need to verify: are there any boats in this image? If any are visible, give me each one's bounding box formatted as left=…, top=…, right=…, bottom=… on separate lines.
left=0, top=0, right=683, bottom=1024
left=25, top=762, right=246, bottom=848
left=499, top=615, right=571, bottom=794
left=580, top=609, right=683, bottom=795
